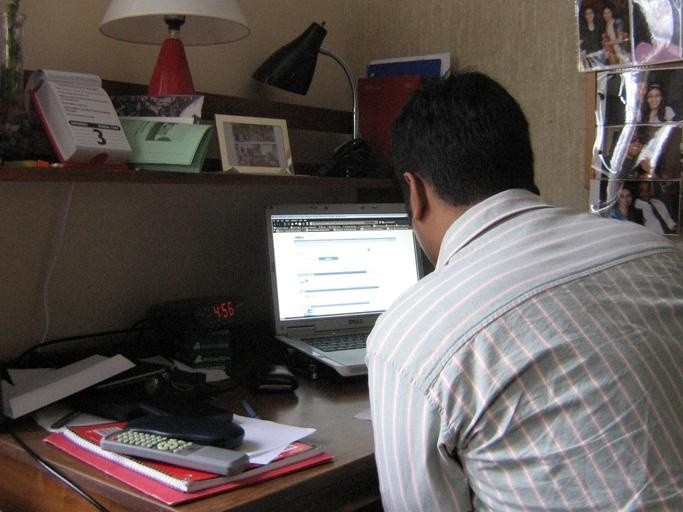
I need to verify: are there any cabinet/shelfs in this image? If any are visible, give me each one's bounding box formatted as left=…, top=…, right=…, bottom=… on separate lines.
left=0, top=67, right=403, bottom=198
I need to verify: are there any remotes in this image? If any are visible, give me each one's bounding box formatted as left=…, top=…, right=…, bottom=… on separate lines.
left=100, top=428, right=250, bottom=476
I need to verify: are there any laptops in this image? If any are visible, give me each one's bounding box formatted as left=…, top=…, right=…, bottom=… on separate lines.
left=267, top=204, right=426, bottom=380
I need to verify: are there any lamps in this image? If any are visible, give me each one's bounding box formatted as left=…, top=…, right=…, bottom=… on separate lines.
left=252, top=21, right=396, bottom=180
left=99, top=0, right=251, bottom=95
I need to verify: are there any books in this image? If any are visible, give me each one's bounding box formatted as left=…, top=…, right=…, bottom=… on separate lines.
left=62, top=416, right=322, bottom=493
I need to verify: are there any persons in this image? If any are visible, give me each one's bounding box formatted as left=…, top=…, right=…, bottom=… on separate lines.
left=609, top=187, right=634, bottom=223
left=636, top=85, right=676, bottom=125
left=620, top=128, right=644, bottom=177
left=601, top=7, right=625, bottom=64
left=366, top=70, right=683, bottom=511
left=579, top=6, right=601, bottom=68
left=635, top=182, right=677, bottom=234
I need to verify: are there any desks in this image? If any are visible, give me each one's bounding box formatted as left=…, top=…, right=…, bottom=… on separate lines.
left=1, top=328, right=404, bottom=512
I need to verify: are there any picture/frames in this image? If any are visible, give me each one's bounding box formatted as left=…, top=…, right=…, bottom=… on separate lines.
left=214, top=113, right=296, bottom=175
